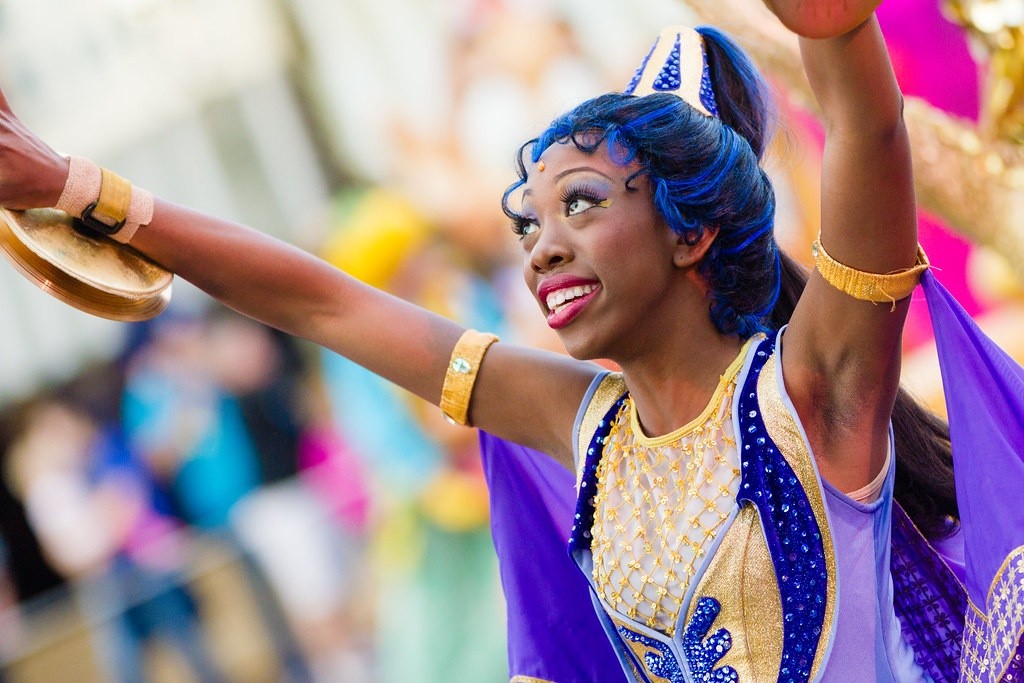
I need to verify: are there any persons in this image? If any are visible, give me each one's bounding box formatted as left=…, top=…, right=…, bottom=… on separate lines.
left=0, top=198, right=503, bottom=683
left=0, top=0, right=1024, bottom=683
